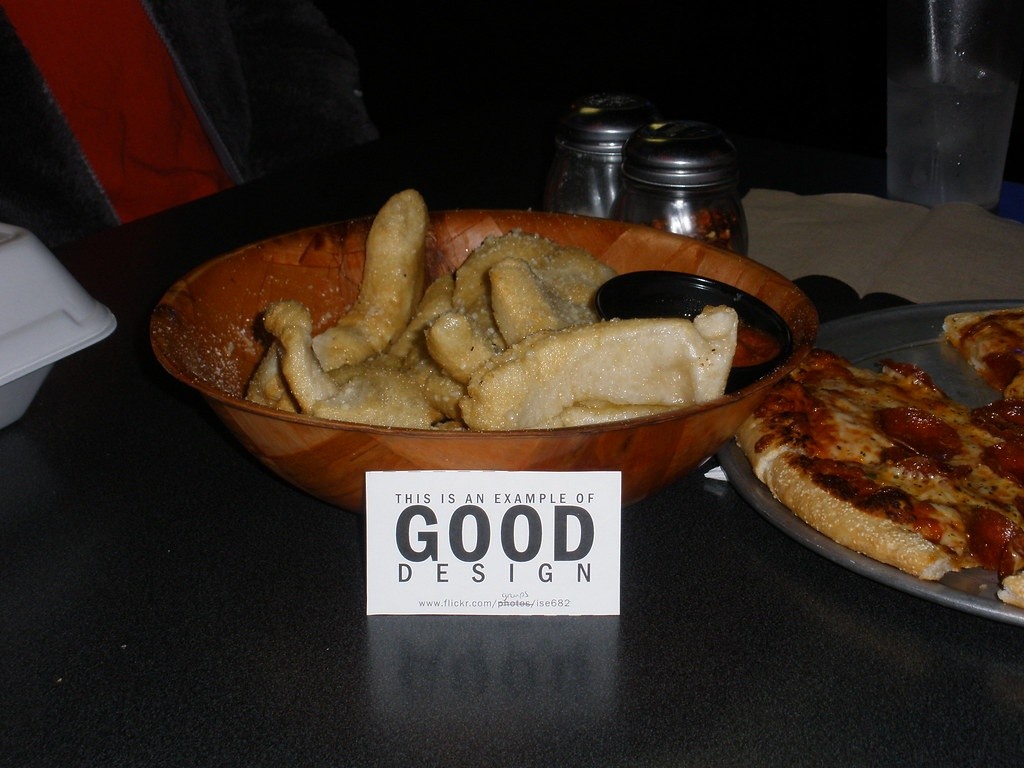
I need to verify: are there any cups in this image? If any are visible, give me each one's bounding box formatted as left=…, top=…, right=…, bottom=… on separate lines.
left=886, top=0, right=1018, bottom=208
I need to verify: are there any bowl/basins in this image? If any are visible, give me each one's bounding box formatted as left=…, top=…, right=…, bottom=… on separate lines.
left=150, top=211, right=818, bottom=510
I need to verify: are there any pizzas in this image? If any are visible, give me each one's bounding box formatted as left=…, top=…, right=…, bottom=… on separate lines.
left=737, top=307, right=1024, bottom=612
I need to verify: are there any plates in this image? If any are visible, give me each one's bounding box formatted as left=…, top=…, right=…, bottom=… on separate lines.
left=717, top=298, right=1024, bottom=624
left=595, top=270, right=794, bottom=392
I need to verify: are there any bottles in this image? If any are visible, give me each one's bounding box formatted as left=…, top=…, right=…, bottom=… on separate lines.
left=546, top=92, right=656, bottom=220
left=607, top=120, right=748, bottom=260
